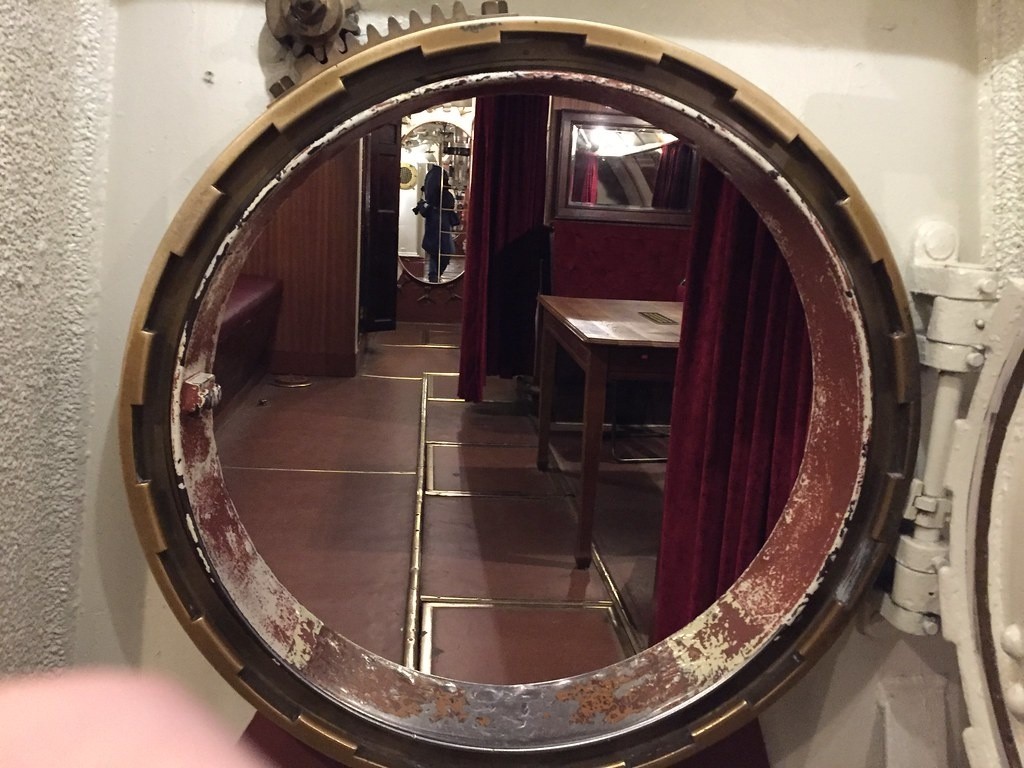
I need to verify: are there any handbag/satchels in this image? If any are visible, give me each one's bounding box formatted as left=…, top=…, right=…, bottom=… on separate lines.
left=450, top=212, right=460, bottom=226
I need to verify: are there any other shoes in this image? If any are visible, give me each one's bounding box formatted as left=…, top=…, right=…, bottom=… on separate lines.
left=428, top=272, right=443, bottom=282
left=454, top=240, right=465, bottom=255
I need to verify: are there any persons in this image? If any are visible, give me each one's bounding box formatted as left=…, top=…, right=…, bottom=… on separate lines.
left=422, top=166, right=461, bottom=282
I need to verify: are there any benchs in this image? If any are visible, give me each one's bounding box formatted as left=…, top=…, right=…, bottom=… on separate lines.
left=211, top=273, right=283, bottom=417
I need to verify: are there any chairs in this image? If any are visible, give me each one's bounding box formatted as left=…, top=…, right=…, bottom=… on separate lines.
left=605, top=347, right=675, bottom=464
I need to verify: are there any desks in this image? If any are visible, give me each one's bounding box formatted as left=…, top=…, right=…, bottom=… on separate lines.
left=538, top=294, right=683, bottom=572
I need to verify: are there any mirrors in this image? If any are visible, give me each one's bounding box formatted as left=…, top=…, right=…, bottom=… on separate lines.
left=551, top=107, right=700, bottom=229
